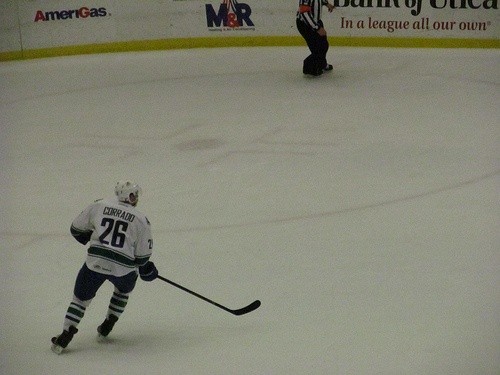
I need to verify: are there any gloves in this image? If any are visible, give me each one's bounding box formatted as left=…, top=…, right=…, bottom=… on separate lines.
left=139, top=261, right=158, bottom=282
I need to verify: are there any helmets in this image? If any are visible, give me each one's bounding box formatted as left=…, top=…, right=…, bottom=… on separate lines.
left=115, top=180, right=140, bottom=202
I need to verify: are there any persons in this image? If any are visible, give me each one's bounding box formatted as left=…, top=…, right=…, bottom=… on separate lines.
left=294, top=0, right=335, bottom=78
left=50, top=180, right=159, bottom=355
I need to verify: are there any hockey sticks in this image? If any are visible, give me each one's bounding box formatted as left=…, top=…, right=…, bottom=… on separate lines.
left=157, top=275, right=261, bottom=315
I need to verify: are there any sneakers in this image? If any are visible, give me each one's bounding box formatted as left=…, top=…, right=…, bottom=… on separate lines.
left=97, top=313, right=118, bottom=343
left=323, top=64, right=333, bottom=71
left=51, top=325, right=78, bottom=354
